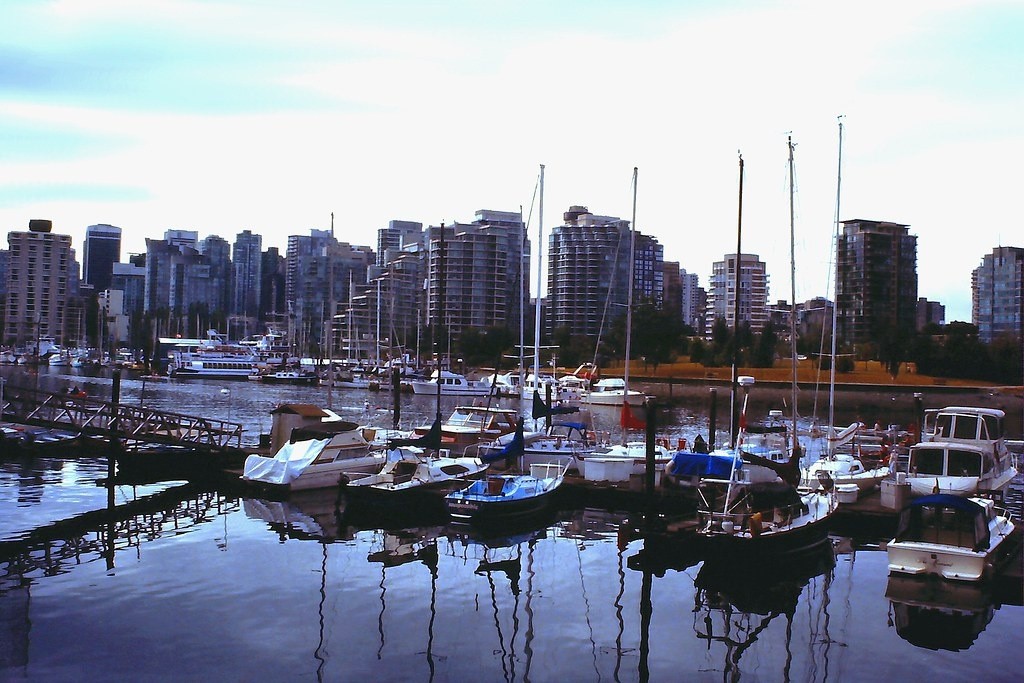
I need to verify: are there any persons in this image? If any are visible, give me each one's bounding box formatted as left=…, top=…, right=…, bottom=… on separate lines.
left=71, top=385, right=80, bottom=395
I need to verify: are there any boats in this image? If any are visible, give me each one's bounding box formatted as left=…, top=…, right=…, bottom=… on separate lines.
left=886, top=477, right=1015, bottom=583
left=884, top=569, right=1002, bottom=652
left=0, top=336, right=152, bottom=370
left=240, top=421, right=388, bottom=495
left=487, top=363, right=645, bottom=406
left=904, top=406, right=1018, bottom=506
left=660, top=364, right=793, bottom=487
left=411, top=369, right=498, bottom=396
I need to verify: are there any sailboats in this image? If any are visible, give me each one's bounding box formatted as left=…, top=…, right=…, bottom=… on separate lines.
left=695, top=136, right=840, bottom=563
left=442, top=204, right=574, bottom=522
left=337, top=223, right=491, bottom=514
left=413, top=168, right=694, bottom=487
left=167, top=270, right=431, bottom=392
left=694, top=538, right=836, bottom=683
left=797, top=122, right=894, bottom=500
